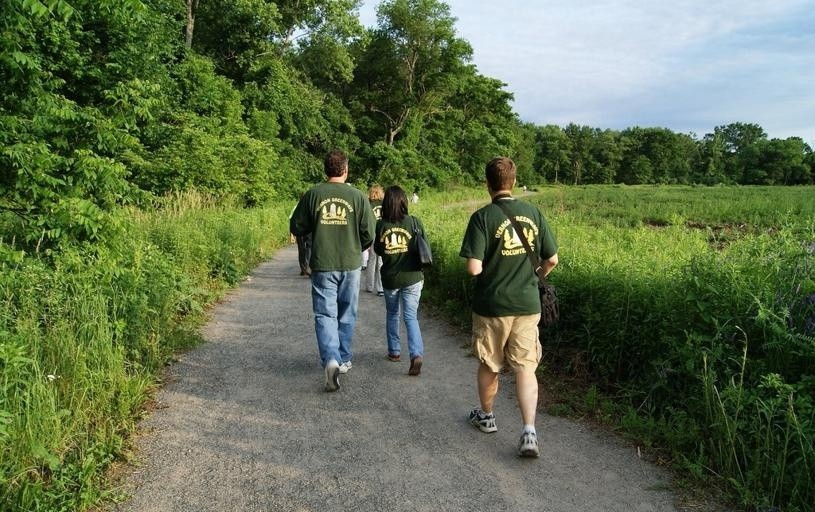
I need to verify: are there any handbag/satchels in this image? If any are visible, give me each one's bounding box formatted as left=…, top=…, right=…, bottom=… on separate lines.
left=538, top=282, right=561, bottom=328
left=407, top=229, right=434, bottom=272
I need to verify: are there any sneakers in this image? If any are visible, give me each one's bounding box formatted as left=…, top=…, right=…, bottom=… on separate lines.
left=300, top=268, right=312, bottom=276
left=339, top=360, right=353, bottom=374
left=376, top=291, right=384, bottom=297
left=517, top=430, right=541, bottom=459
left=324, top=359, right=340, bottom=393
left=408, top=355, right=423, bottom=376
left=387, top=352, right=401, bottom=362
left=469, top=408, right=499, bottom=434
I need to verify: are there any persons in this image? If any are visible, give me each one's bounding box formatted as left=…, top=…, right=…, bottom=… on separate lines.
left=288, top=192, right=312, bottom=277
left=459, top=156, right=560, bottom=457
left=365, top=183, right=386, bottom=297
left=288, top=150, right=379, bottom=392
left=373, top=185, right=430, bottom=376
left=411, top=192, right=419, bottom=203
left=362, top=247, right=371, bottom=270
